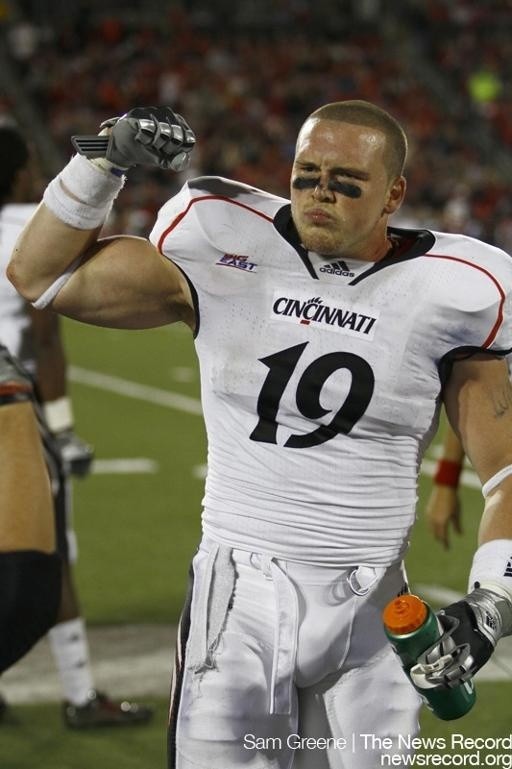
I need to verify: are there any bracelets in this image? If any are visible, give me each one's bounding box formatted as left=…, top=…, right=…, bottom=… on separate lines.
left=40, top=396, right=74, bottom=433
left=433, top=459, right=461, bottom=489
left=42, top=152, right=125, bottom=231
left=467, top=539, right=512, bottom=596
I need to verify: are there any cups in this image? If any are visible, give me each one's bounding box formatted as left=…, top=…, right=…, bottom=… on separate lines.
left=382, top=594, right=476, bottom=721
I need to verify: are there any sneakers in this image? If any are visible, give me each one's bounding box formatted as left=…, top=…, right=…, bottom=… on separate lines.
left=63, top=694, right=152, bottom=728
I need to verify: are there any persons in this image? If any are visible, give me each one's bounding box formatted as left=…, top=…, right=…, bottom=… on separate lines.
left=6, top=100, right=512, bottom=769
left=0, top=125, right=153, bottom=729
left=426, top=420, right=465, bottom=551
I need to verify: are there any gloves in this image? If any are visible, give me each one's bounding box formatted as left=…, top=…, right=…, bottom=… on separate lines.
left=386, top=589, right=512, bottom=692
left=56, top=429, right=93, bottom=477
left=71, top=105, right=196, bottom=178
